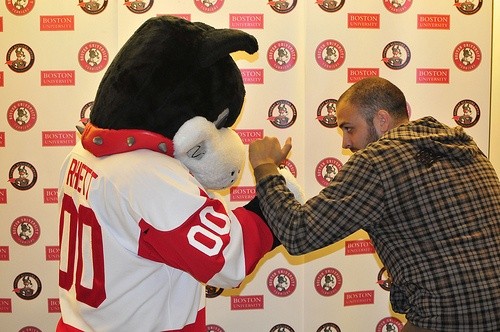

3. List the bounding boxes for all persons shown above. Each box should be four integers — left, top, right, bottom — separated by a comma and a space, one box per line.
249, 77, 500, 332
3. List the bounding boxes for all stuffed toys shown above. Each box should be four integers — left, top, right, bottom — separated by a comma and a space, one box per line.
56, 14, 304, 332
